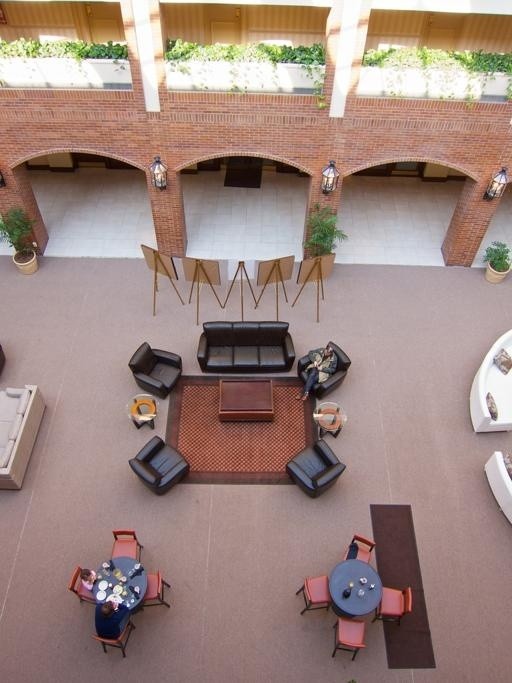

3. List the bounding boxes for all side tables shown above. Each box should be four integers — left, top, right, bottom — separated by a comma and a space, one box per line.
312, 401, 347, 438
126, 394, 162, 430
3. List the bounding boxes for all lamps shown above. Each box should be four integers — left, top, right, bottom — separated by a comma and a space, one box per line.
483, 167, 511, 201
321, 161, 341, 194
149, 155, 168, 190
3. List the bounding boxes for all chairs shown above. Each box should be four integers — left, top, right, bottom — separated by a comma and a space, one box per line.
67, 529, 169, 659
129, 342, 182, 400
296, 534, 413, 662
298, 341, 352, 401
286, 439, 347, 498
129, 435, 190, 495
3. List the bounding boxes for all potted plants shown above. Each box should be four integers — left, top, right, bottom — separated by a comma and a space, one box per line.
484, 240, 512, 283
1, 208, 40, 275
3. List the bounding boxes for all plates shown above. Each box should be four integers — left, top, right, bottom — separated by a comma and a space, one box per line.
96, 580, 123, 611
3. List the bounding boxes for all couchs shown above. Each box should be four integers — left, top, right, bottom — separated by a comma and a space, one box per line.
1, 385, 46, 490
198, 321, 296, 372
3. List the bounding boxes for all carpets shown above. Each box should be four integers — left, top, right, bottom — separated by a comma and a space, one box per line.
166, 376, 318, 484
369, 503, 437, 670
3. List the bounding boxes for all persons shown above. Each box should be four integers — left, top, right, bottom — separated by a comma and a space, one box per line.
94, 600, 131, 640
295, 346, 339, 401
79, 568, 98, 591
